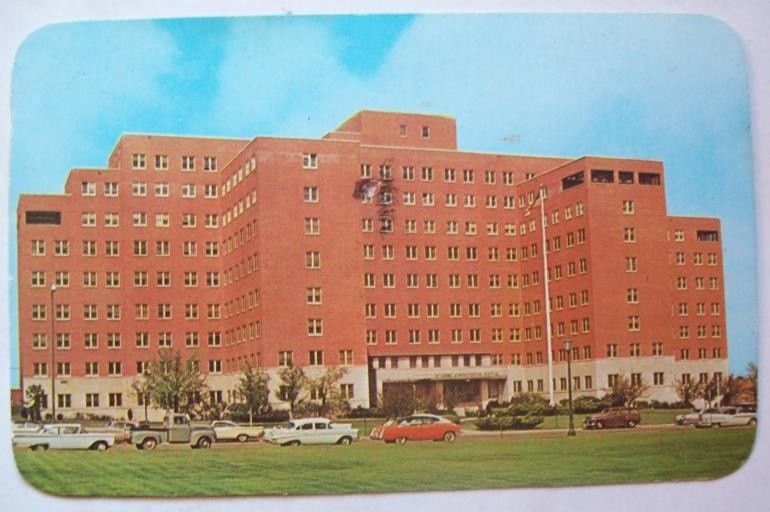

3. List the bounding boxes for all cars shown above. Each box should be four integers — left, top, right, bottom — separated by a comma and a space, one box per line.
12, 423, 115, 452
263, 417, 360, 447
676, 401, 757, 427
82, 419, 141, 445
12, 421, 65, 435
370, 413, 461, 446
206, 418, 266, 442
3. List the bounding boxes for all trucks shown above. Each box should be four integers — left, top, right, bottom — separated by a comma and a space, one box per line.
129, 414, 217, 451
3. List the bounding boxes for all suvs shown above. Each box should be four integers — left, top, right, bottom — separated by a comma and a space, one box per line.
584, 406, 641, 430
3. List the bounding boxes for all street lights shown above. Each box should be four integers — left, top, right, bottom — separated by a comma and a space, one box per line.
50, 282, 58, 422
563, 336, 578, 438
522, 181, 556, 407
